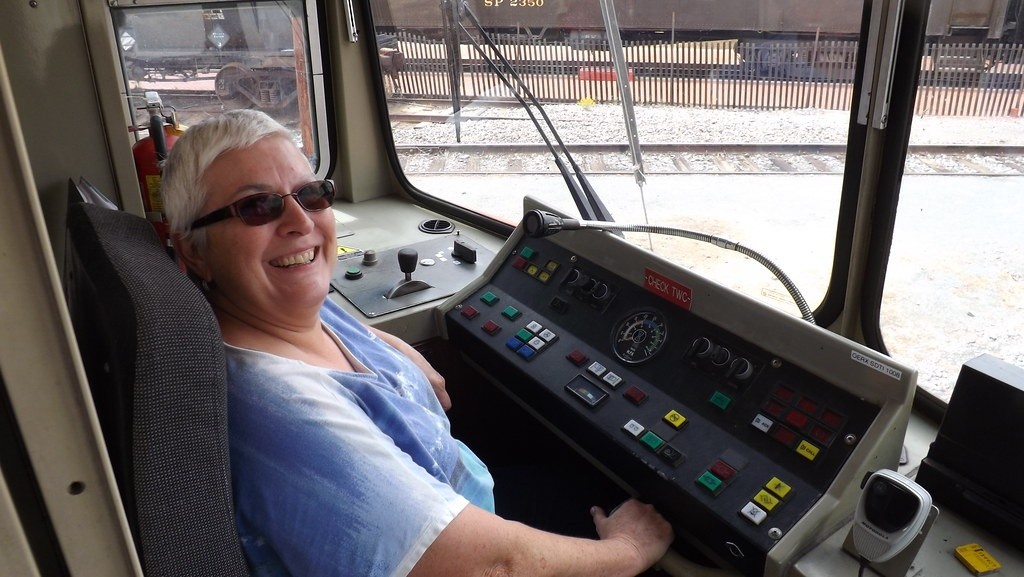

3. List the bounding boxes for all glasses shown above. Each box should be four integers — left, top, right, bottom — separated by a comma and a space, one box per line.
185, 179, 336, 232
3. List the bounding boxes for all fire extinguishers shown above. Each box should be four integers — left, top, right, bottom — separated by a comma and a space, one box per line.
122, 91, 186, 256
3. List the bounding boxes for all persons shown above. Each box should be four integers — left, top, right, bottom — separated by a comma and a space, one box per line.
162, 109, 674, 577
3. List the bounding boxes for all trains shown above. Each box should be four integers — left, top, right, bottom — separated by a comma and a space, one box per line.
114, 0, 402, 115
372, 0, 1024, 77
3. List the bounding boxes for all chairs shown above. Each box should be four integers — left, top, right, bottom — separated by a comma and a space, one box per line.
65, 202, 252, 577
